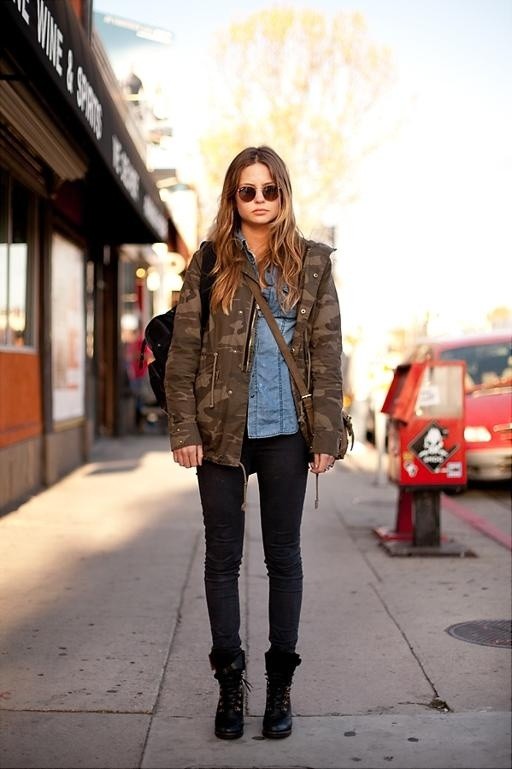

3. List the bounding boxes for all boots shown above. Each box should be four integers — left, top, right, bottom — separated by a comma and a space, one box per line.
208, 645, 254, 739
261, 651, 303, 739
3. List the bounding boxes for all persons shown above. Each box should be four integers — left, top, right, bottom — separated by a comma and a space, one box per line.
163, 142, 343, 740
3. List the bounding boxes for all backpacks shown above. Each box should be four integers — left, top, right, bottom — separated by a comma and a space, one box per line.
143, 240, 217, 413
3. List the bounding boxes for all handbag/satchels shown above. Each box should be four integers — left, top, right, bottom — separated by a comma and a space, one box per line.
298, 393, 355, 461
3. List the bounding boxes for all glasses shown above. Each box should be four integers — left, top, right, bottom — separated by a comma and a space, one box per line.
235, 185, 279, 202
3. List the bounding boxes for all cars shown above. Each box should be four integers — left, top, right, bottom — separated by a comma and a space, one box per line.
409, 334, 512, 496
